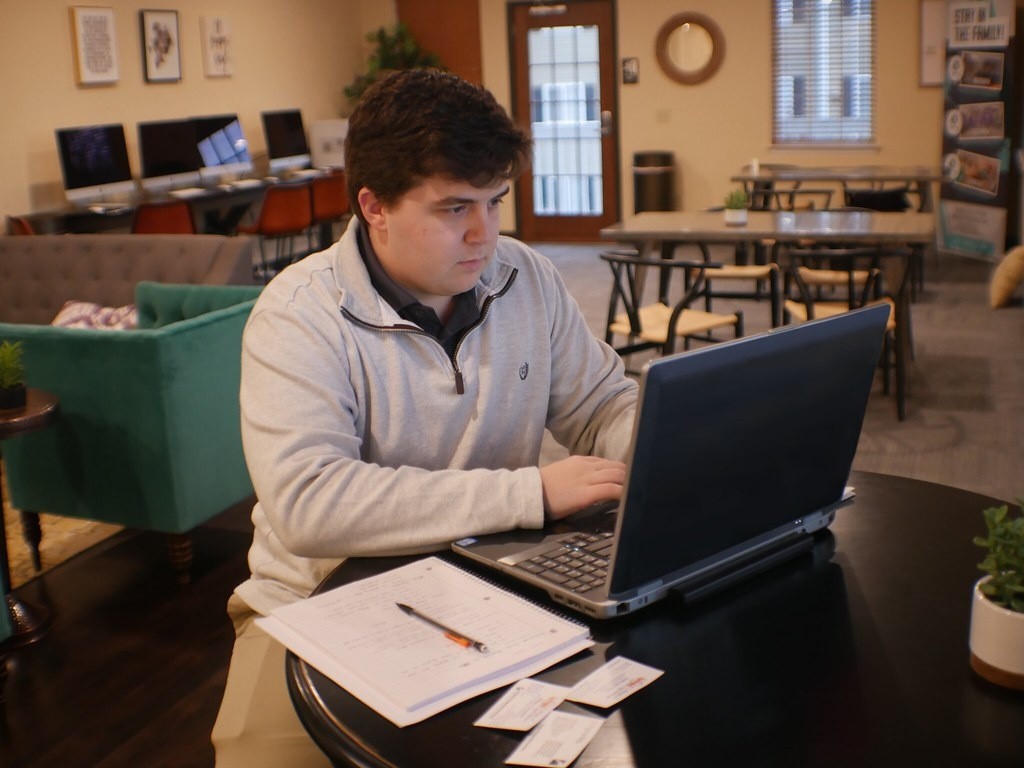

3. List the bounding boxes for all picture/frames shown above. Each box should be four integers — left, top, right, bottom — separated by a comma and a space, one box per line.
141, 9, 183, 84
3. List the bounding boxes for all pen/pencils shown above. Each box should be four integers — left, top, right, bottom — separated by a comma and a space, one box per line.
395, 603, 487, 652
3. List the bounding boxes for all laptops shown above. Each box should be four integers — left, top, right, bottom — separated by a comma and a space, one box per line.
449, 303, 892, 620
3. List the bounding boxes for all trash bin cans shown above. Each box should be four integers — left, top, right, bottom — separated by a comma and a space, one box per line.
632, 151, 673, 215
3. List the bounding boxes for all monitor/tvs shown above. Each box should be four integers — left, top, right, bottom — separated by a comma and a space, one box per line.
54, 109, 312, 202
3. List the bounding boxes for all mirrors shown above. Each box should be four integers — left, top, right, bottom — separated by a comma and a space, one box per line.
656, 9, 726, 86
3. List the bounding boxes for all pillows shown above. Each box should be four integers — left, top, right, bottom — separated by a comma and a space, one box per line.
989, 243, 1024, 307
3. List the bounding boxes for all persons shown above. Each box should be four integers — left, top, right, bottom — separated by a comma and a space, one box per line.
211, 67, 640, 768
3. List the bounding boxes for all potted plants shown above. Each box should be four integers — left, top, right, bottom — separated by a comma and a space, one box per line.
724, 189, 748, 225
965, 501, 1024, 690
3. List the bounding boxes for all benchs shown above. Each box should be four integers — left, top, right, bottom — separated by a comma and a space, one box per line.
3, 229, 256, 329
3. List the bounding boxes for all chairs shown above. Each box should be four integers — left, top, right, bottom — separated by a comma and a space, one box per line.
0, 282, 272, 585
130, 200, 198, 236
597, 160, 929, 423
235, 182, 314, 281
301, 173, 353, 261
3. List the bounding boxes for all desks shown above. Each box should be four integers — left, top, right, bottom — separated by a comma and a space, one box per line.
60, 164, 346, 236
600, 209, 935, 420
728, 168, 933, 292
283, 446, 1024, 766
1, 386, 63, 651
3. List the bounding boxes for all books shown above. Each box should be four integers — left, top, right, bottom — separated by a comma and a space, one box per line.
252, 557, 595, 728
